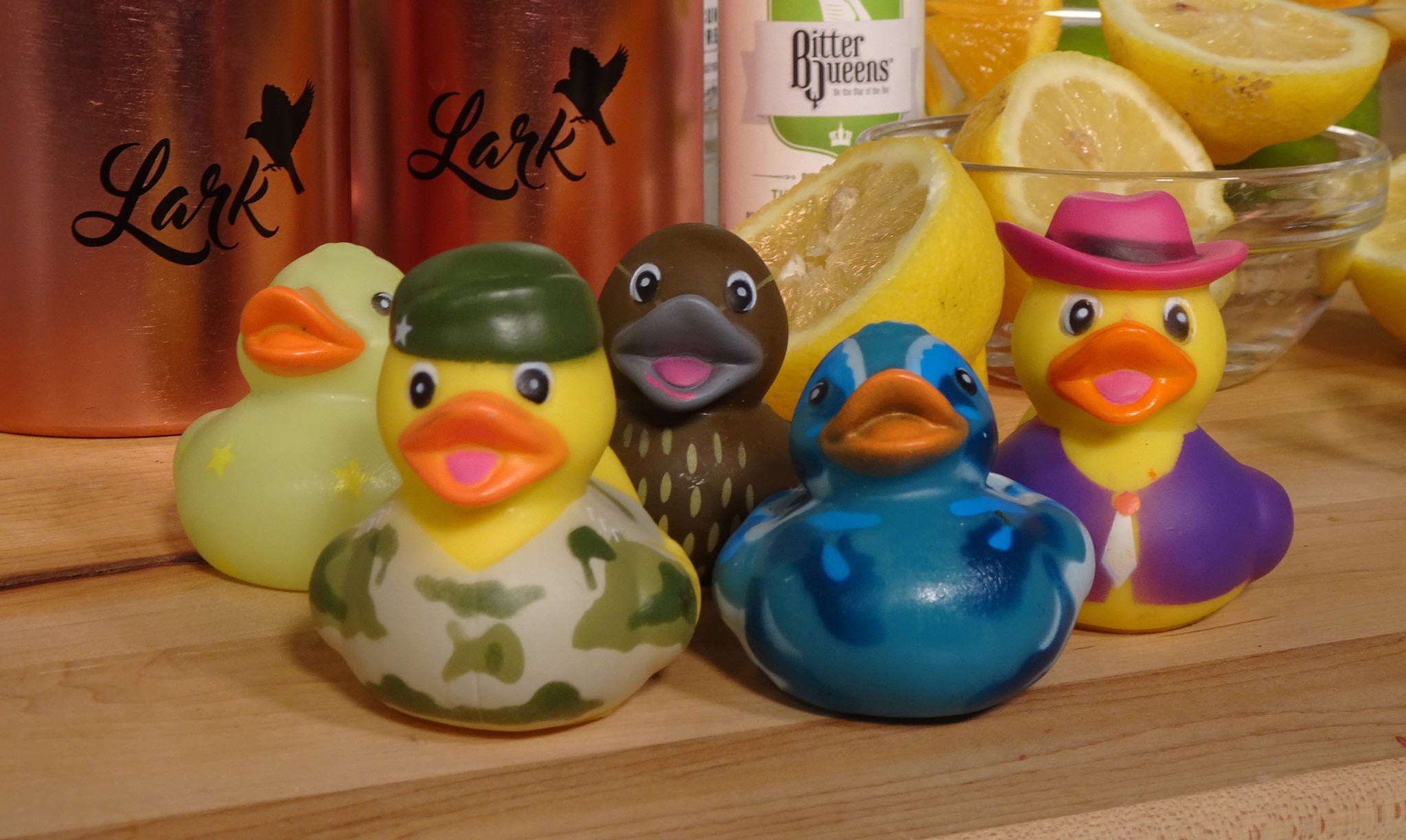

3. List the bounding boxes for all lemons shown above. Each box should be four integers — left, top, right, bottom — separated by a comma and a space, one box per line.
918, 1, 1389, 332
732, 134, 1006, 426
1349, 150, 1406, 343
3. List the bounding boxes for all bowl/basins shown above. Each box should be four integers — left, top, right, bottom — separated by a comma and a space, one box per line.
853, 111, 1392, 394
